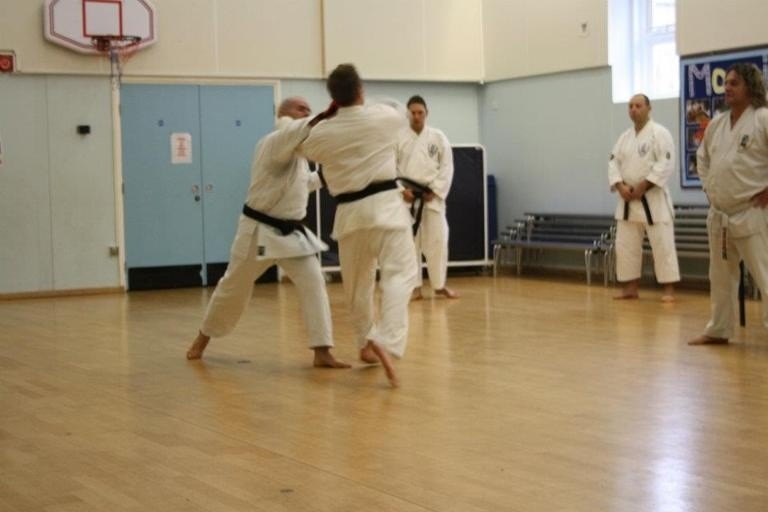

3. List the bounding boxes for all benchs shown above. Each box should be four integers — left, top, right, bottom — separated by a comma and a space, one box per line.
491, 203, 759, 299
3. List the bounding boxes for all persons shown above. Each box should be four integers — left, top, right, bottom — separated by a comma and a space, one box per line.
186, 97, 352, 369
278, 63, 412, 388
394, 95, 461, 299
607, 93, 682, 302
687, 98, 712, 121
686, 62, 767, 345
